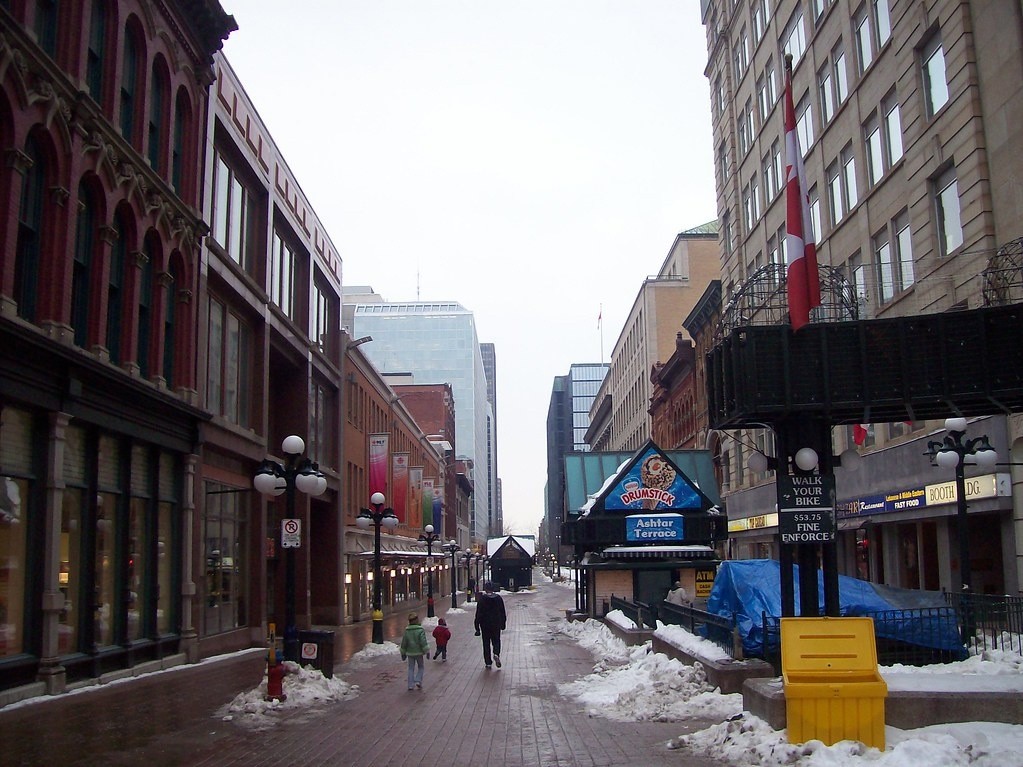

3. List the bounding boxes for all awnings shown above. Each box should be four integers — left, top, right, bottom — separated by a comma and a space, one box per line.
357, 551, 445, 559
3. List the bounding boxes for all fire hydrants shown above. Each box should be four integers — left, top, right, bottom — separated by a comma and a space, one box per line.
264, 647, 289, 702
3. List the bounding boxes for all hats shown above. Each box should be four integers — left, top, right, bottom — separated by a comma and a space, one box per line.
408, 611, 419, 624
670, 581, 678, 591
438, 618, 446, 625
485, 581, 494, 591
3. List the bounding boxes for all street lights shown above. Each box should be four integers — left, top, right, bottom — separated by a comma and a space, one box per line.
556, 516, 561, 578
462, 548, 489, 604
927, 418, 998, 647
416, 525, 442, 618
254, 436, 327, 664
444, 540, 463, 609
357, 492, 400, 645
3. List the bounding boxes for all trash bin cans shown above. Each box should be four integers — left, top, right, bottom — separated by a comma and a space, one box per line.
779, 616, 888, 754
297, 629, 335, 679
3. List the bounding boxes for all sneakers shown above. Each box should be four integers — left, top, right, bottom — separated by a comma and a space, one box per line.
494, 654, 501, 668
484, 665, 491, 668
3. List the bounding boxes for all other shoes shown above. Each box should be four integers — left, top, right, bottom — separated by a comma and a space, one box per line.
443, 659, 447, 662
408, 687, 413, 690
416, 682, 422, 688
433, 656, 436, 660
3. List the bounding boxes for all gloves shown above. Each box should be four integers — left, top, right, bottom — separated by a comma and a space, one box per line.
686, 599, 690, 603
402, 655, 406, 661
426, 653, 430, 659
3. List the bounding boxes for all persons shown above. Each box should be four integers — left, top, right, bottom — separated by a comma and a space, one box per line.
666, 581, 689, 605
474, 582, 506, 670
469, 576, 475, 594
432, 618, 451, 663
399, 612, 430, 691
